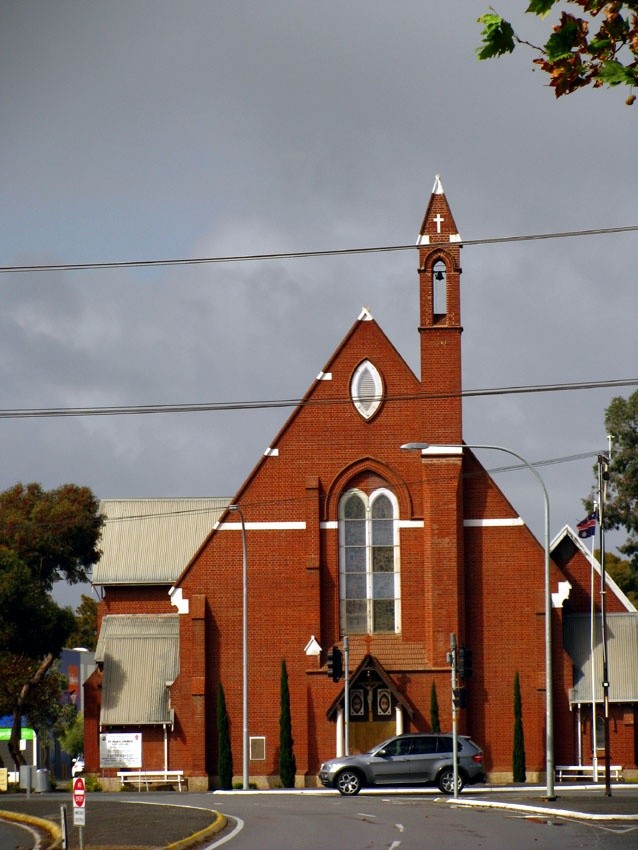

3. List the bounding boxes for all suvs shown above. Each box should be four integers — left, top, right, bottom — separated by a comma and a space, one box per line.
319, 732, 488, 795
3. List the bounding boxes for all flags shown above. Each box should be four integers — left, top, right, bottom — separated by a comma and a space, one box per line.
576, 511, 596, 538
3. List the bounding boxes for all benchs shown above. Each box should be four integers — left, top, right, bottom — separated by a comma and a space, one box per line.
117, 770, 185, 792
555, 766, 622, 781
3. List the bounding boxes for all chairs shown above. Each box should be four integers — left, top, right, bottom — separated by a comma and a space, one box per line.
398, 741, 416, 754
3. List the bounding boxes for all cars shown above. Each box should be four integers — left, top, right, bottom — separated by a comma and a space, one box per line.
72, 755, 85, 776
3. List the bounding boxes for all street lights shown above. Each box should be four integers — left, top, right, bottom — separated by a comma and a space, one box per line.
401, 442, 555, 797
230, 505, 249, 791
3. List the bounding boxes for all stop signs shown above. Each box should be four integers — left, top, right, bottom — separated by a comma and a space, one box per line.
73, 776, 85, 807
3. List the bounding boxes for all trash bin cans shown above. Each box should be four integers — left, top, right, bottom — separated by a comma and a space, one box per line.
35, 768, 49, 793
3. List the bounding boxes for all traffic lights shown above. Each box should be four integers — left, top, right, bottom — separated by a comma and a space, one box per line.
326, 647, 342, 681
459, 648, 474, 677
453, 685, 468, 709
